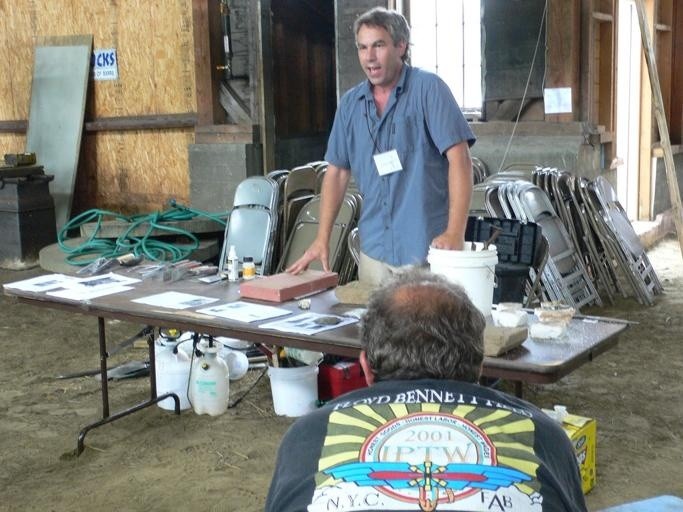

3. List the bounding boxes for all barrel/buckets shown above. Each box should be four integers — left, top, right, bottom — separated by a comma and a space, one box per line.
189, 349, 230, 416
155, 332, 192, 412
263, 363, 321, 417
427, 240, 500, 317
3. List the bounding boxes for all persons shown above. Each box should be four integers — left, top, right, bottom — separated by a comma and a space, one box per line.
263, 268, 589, 510
282, 6, 479, 287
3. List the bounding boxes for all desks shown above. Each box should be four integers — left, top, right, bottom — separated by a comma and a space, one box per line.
3, 264, 630, 461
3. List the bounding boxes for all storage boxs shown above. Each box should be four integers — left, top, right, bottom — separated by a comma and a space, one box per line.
540, 407, 596, 495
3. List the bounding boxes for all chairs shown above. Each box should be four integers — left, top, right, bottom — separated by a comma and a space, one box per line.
213, 157, 662, 315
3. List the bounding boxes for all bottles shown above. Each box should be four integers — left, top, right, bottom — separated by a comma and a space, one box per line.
227, 246, 239, 281
243, 257, 255, 279
